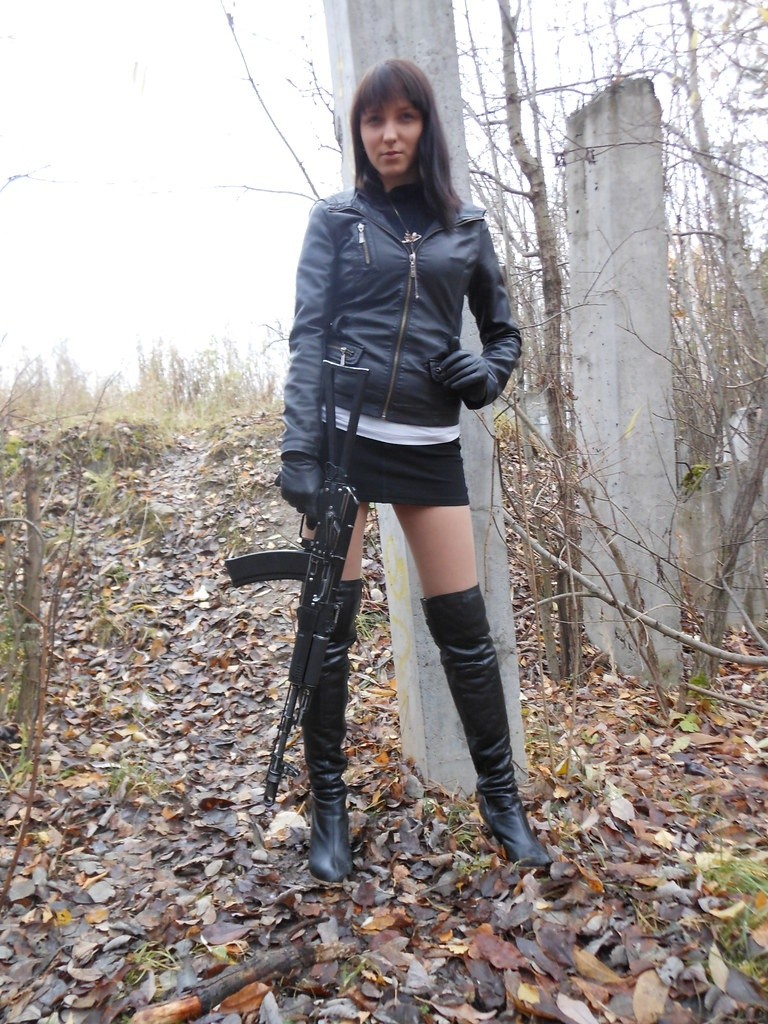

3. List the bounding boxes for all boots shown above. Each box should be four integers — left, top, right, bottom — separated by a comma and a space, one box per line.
297, 579, 363, 883
420, 582, 554, 870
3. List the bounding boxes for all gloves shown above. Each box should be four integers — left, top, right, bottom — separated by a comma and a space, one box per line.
281, 452, 327, 530
437, 349, 486, 407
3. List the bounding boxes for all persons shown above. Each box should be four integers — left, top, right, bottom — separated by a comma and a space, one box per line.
277, 60, 552, 879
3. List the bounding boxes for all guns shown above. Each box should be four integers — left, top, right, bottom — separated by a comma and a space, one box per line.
220, 358, 374, 810
